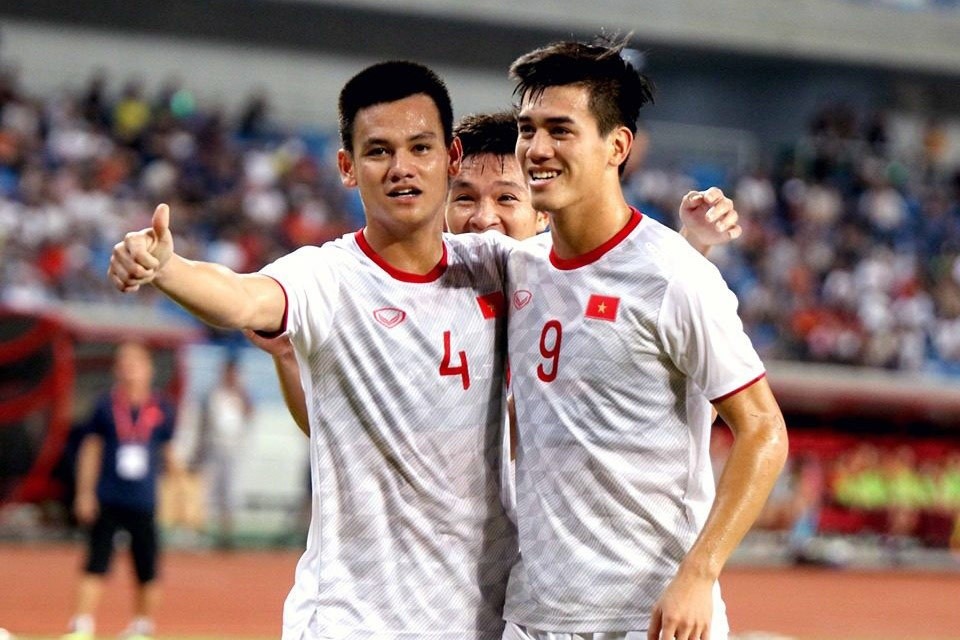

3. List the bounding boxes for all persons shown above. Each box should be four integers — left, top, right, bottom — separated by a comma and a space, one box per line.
0, 63, 960, 384
443, 33, 788, 640
241, 107, 550, 514
60, 336, 189, 640
107, 57, 741, 640
190, 356, 254, 555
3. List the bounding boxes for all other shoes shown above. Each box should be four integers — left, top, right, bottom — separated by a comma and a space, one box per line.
124, 619, 153, 640
65, 613, 96, 640
